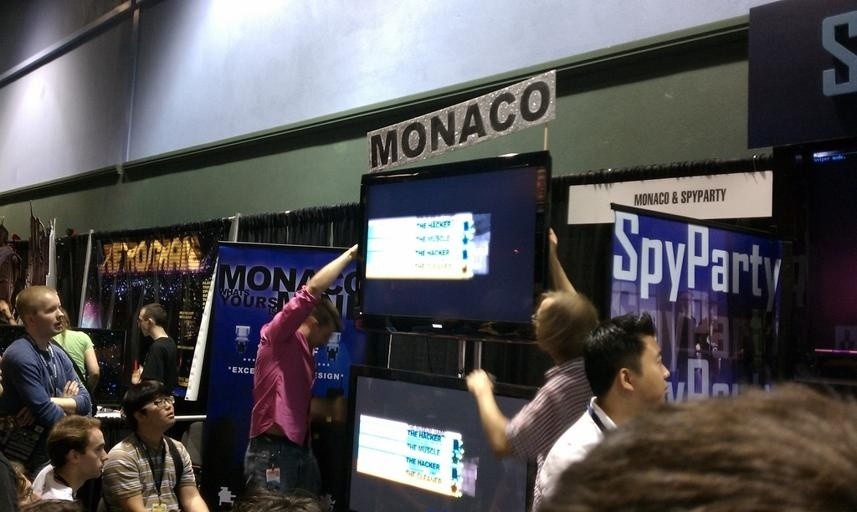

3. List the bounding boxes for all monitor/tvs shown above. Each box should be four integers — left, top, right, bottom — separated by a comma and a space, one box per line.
342, 364, 542, 512
353, 149, 552, 344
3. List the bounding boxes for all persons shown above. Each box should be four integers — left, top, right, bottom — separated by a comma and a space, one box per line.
735, 318, 759, 382
700, 302, 721, 358
230, 487, 338, 511
538, 312, 673, 506
464, 226, 602, 510
4, 284, 211, 511
241, 239, 360, 491
537, 383, 855, 511
